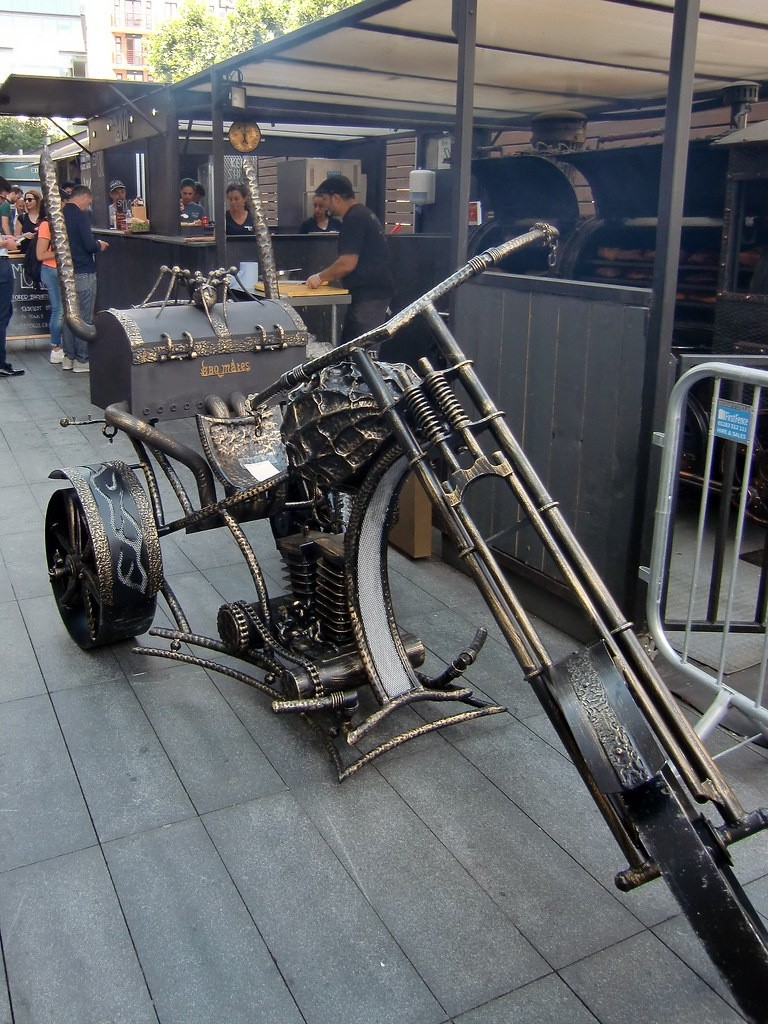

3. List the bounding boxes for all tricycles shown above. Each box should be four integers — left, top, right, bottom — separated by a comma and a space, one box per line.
37, 144, 768, 1024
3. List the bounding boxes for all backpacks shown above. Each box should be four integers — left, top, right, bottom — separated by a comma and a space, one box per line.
22, 219, 51, 283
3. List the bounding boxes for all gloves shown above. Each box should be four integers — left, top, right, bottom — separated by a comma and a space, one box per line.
305, 273, 322, 289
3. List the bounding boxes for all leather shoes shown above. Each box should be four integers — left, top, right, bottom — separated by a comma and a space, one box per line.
0, 363, 25, 377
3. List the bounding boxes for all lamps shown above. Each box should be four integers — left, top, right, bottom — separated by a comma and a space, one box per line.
224, 69, 246, 110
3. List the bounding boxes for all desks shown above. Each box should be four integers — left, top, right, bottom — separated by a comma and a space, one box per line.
231, 280, 352, 349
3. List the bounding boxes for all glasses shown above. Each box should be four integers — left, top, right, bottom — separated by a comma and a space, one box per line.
24, 198, 35, 203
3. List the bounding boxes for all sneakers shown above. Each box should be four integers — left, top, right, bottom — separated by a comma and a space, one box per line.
50, 349, 64, 363
62, 356, 74, 370
72, 359, 90, 372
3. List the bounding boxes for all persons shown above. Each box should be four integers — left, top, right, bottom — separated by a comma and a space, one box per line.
316, 174, 395, 364
225, 183, 255, 235
300, 194, 342, 235
2, 174, 207, 371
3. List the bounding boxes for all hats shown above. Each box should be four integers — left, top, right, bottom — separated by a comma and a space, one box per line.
109, 179, 125, 192
313, 175, 353, 194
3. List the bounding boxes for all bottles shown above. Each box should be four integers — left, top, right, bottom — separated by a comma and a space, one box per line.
125, 210, 133, 230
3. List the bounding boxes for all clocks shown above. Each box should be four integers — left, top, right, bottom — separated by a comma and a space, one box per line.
229, 118, 261, 153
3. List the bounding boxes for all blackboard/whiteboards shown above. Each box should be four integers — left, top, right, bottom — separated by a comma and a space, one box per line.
5, 254, 62, 340
